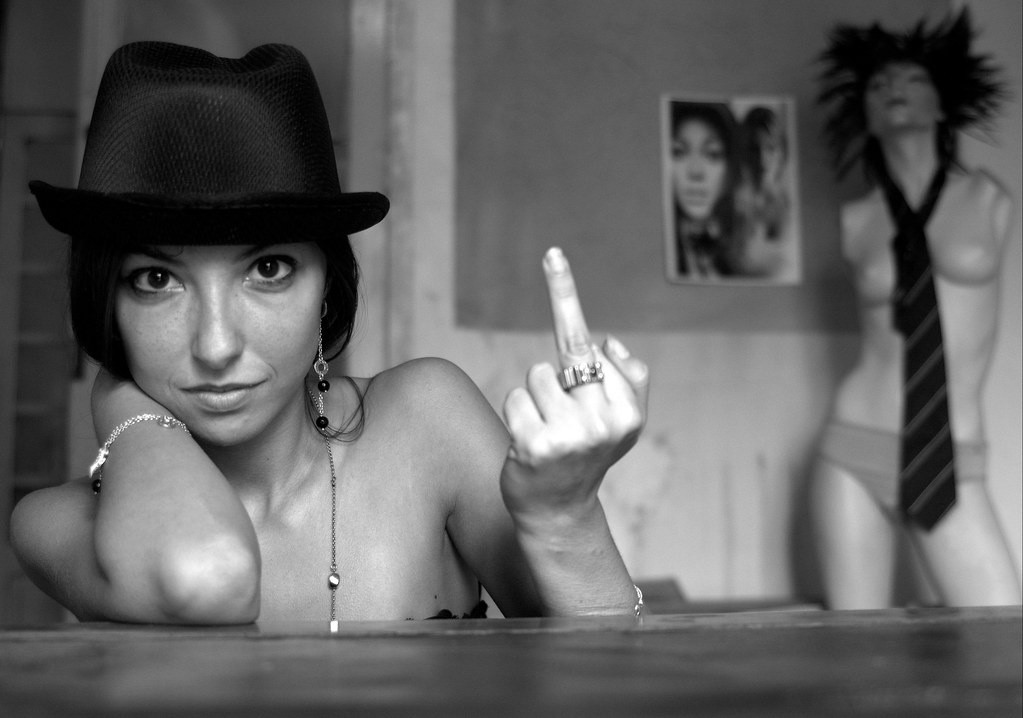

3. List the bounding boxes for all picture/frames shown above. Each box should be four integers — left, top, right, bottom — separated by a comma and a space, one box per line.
660, 92, 801, 289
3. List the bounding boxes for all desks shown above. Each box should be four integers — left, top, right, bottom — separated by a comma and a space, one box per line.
0, 608, 1023, 718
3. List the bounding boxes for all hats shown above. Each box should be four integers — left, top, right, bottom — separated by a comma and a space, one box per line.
28, 40, 391, 245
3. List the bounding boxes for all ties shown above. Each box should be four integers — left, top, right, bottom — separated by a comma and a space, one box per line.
881, 158, 958, 533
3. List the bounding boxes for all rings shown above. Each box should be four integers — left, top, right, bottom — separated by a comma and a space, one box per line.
558, 362, 605, 389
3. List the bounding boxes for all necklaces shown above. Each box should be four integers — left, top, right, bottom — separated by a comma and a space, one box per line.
309, 387, 339, 619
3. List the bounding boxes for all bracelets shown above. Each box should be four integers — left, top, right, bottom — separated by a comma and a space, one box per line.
89, 415, 192, 496
634, 584, 643, 615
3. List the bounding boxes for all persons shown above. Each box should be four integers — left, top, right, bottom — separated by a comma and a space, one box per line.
10, 37, 651, 626
669, 102, 792, 281
810, 9, 1019, 610
